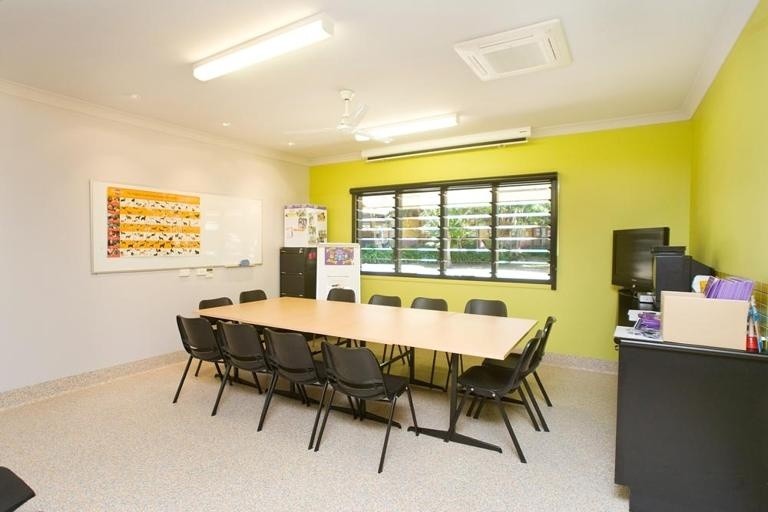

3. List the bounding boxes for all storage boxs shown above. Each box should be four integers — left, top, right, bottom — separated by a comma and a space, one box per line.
659, 290, 749, 353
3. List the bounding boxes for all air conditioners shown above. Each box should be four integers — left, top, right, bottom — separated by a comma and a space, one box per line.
360, 125, 532, 167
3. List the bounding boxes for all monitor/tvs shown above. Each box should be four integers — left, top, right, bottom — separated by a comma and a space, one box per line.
611, 227, 670, 296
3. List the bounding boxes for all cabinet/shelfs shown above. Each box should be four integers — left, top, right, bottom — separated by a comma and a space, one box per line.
280, 247, 316, 300
317, 243, 360, 306
613, 306, 768, 512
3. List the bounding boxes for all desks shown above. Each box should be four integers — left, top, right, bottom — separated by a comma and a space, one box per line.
193, 296, 538, 455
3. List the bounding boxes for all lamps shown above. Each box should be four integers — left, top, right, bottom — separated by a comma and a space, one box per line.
192, 17, 334, 79
346, 118, 459, 143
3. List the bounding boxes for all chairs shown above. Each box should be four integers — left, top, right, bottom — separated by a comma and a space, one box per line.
194, 296, 232, 386
410, 298, 449, 310
464, 298, 504, 316
257, 327, 357, 451
173, 313, 234, 403
370, 294, 400, 306
237, 289, 266, 302
446, 330, 545, 466
209, 321, 306, 432
316, 340, 419, 478
328, 289, 354, 304
466, 316, 559, 437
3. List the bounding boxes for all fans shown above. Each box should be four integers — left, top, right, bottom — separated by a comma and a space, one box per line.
293, 99, 393, 147
